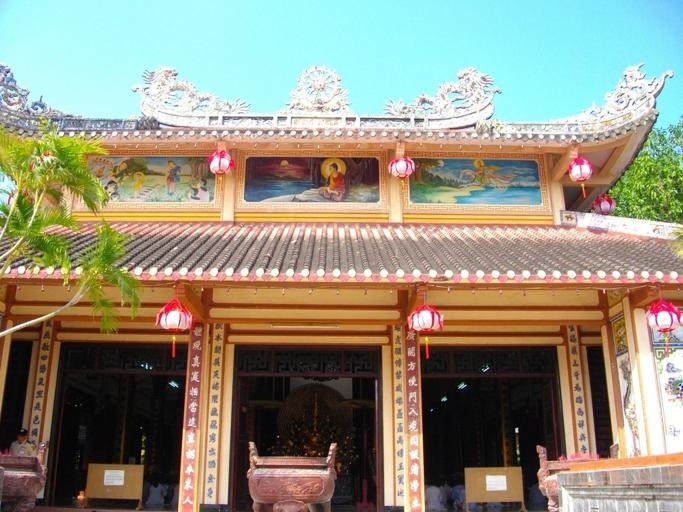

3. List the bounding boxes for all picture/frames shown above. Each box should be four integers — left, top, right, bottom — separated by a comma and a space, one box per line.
403, 146, 554, 215
230, 141, 392, 214
76, 142, 224, 212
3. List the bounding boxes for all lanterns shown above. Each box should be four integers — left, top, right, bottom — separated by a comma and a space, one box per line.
592, 194, 615, 215
407, 304, 444, 360
387, 157, 416, 191
30, 152, 61, 179
208, 151, 236, 188
154, 298, 193, 358
567, 157, 594, 201
646, 300, 682, 355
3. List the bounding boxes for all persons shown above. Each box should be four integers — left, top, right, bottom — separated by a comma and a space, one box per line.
424, 480, 546, 512
264, 391, 358, 475
10, 429, 37, 455
143, 479, 178, 510
